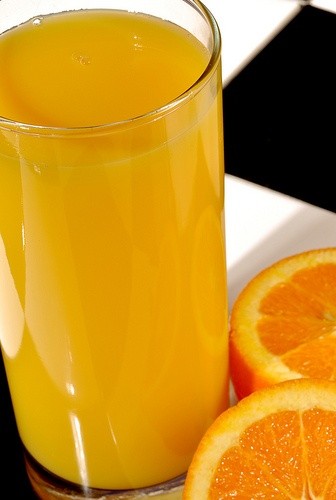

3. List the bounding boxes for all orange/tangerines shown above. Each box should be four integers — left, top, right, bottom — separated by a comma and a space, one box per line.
229, 247, 336, 402
182, 377, 336, 500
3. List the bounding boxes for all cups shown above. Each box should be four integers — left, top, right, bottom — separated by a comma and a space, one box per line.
0, 0, 231, 500
